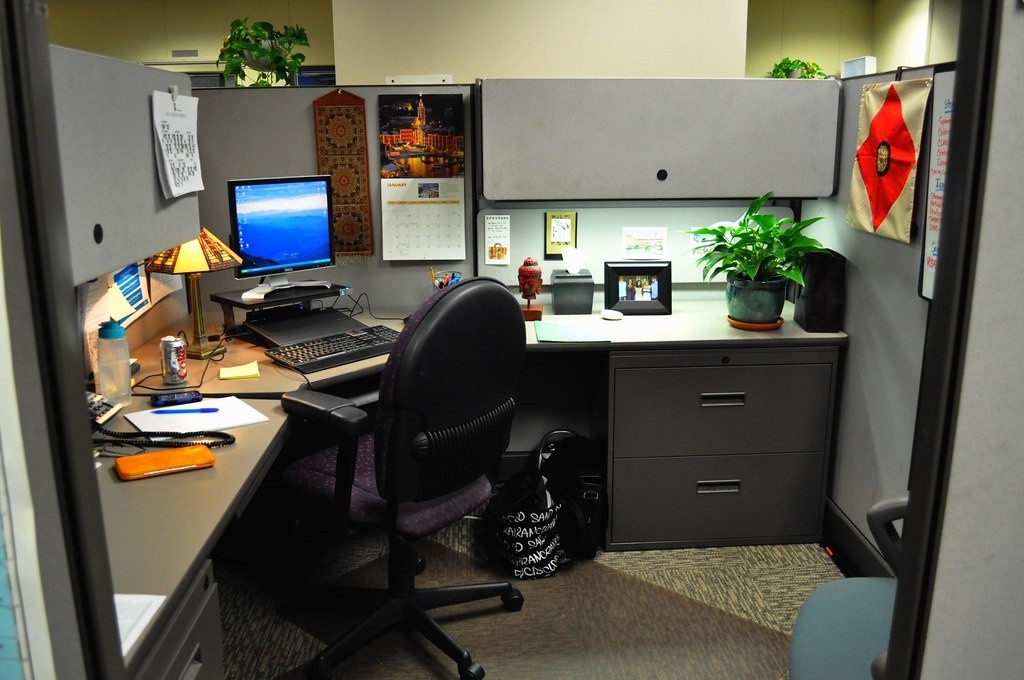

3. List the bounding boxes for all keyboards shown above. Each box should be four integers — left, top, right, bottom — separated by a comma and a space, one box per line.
263, 325, 402, 374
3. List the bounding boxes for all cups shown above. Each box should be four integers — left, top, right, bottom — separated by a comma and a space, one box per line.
432, 271, 463, 295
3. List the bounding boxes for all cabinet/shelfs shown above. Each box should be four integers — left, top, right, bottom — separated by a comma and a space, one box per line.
196, 291, 850, 553
82, 315, 313, 680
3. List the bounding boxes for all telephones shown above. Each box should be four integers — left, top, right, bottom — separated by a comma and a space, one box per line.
86, 391, 123, 433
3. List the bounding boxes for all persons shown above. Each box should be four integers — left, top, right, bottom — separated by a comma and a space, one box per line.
518, 256, 542, 299
619, 276, 627, 301
650, 275, 658, 300
626, 278, 635, 300
641, 280, 651, 300
634, 280, 643, 301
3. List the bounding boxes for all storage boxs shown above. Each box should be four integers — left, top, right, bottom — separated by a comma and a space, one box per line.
840, 56, 877, 79
551, 269, 594, 315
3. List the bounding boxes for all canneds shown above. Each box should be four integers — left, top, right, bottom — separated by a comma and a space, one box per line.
160, 335, 187, 384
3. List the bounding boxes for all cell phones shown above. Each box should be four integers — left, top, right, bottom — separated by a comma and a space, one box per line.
151, 390, 203, 407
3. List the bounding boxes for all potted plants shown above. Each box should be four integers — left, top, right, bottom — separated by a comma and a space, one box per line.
217, 16, 310, 88
688, 191, 833, 330
766, 58, 825, 80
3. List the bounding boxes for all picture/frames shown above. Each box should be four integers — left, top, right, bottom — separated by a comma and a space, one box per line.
604, 261, 671, 314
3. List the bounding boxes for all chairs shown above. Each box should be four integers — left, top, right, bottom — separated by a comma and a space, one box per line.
259, 278, 525, 680
789, 498, 908, 679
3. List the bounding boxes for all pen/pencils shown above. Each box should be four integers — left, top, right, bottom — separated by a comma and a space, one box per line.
434, 272, 461, 289
150, 408, 219, 415
430, 266, 436, 283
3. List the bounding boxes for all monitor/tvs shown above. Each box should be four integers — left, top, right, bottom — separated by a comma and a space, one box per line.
227, 174, 338, 302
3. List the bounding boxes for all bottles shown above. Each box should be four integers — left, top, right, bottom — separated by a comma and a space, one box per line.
96, 321, 132, 406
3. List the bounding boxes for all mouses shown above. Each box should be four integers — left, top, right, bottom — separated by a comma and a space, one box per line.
403, 315, 414, 324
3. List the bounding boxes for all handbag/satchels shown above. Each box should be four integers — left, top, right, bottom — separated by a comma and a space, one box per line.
541, 435, 607, 561
476, 471, 564, 579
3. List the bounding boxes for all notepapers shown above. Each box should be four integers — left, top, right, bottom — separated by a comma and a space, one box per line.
219, 361, 261, 379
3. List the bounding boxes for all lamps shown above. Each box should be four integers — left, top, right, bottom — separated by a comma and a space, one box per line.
145, 227, 243, 359
